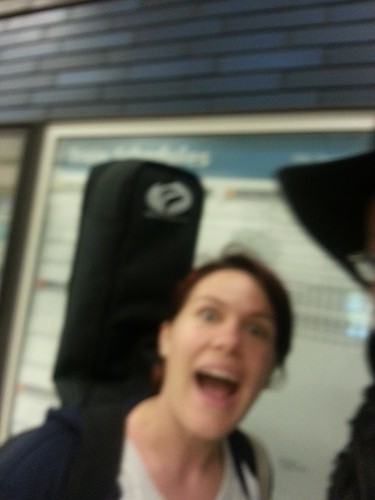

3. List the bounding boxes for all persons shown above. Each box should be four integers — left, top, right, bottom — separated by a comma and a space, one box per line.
0, 243, 293, 499
